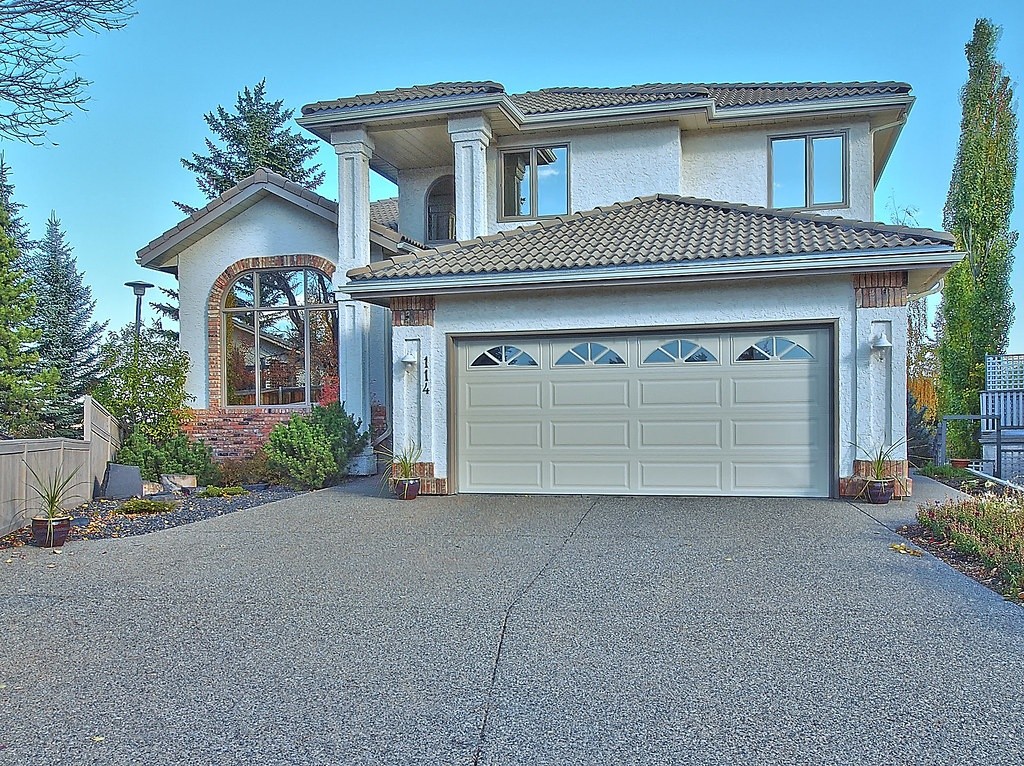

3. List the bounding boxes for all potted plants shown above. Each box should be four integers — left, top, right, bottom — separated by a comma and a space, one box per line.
373, 440, 423, 500
845, 436, 934, 504
1, 455, 97, 547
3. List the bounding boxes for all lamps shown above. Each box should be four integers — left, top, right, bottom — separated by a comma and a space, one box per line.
401, 350, 416, 368
872, 333, 892, 360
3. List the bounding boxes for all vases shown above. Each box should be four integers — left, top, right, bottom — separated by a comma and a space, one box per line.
950, 460, 970, 468
